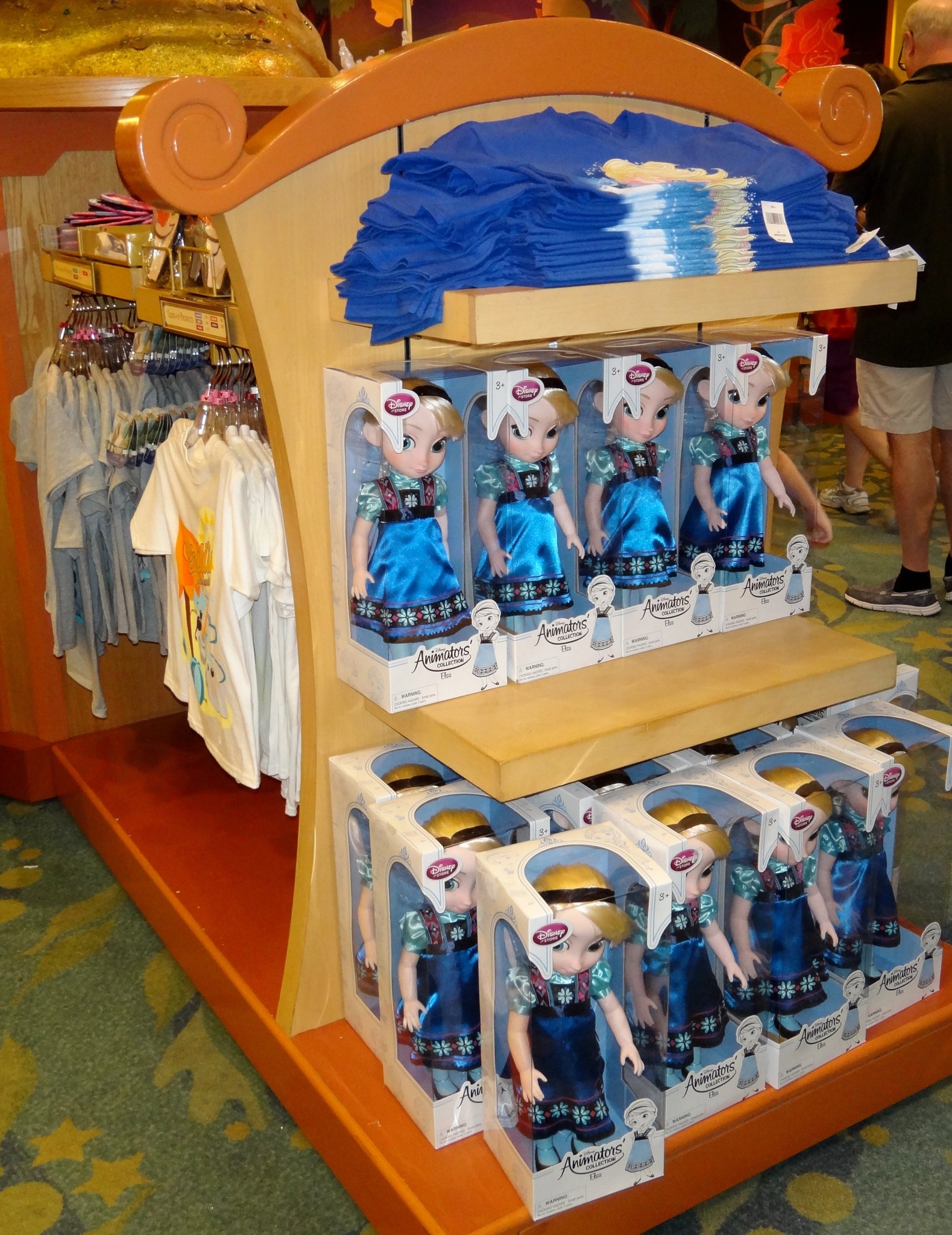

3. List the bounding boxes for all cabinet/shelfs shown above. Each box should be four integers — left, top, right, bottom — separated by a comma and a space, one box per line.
0, 0, 952, 1235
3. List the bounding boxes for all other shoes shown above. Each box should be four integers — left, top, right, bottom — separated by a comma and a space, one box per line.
943, 575, 952, 601
844, 575, 942, 617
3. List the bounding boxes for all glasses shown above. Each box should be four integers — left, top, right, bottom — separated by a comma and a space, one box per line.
897, 30, 913, 72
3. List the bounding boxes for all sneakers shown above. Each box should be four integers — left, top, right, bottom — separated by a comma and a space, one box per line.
820, 483, 871, 514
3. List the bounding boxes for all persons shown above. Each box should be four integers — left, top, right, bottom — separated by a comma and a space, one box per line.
355, 716, 916, 1172
777, 0, 952, 617
579, 352, 677, 609
844, 0, 952, 617
820, 63, 940, 515
355, 764, 446, 997
350, 377, 472, 660
507, 862, 645, 1172
776, 449, 833, 549
394, 807, 505, 1100
677, 343, 795, 587
723, 766, 838, 1040
472, 361, 585, 635
624, 798, 747, 1092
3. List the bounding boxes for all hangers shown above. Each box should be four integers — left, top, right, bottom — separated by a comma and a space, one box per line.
170, 342, 272, 468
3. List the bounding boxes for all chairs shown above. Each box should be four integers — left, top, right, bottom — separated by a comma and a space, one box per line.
38, 294, 139, 400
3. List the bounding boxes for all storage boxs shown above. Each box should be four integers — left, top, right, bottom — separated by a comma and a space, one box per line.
81, 226, 157, 269
328, 325, 952, 1221
38, 224, 83, 258
